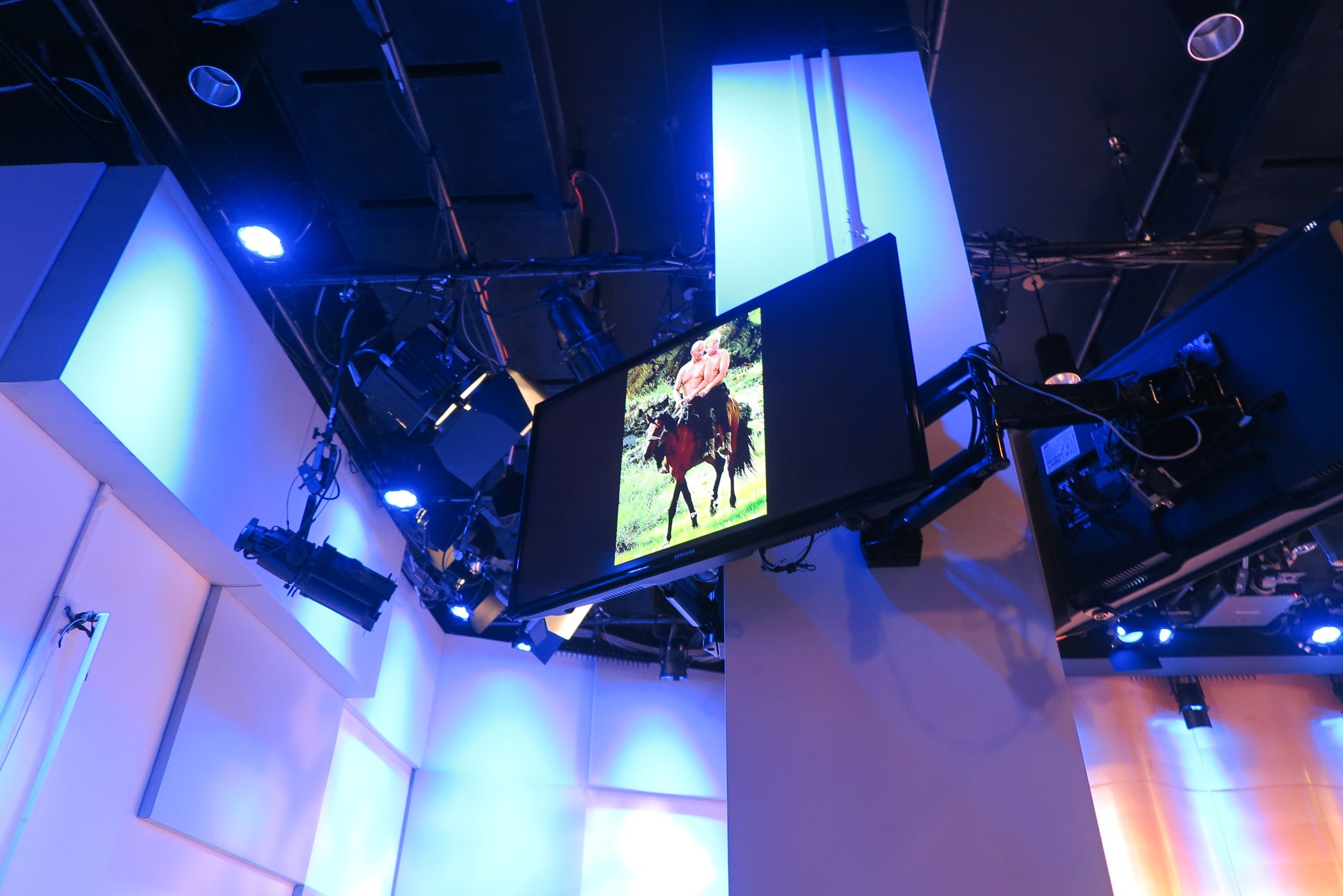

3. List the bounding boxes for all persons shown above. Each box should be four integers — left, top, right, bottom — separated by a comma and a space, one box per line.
672, 331, 733, 460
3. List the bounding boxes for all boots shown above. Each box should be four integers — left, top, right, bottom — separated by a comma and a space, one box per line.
702, 438, 715, 461
720, 432, 733, 455
660, 456, 669, 474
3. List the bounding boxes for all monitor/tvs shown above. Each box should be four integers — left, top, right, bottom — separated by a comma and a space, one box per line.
509, 233, 930, 623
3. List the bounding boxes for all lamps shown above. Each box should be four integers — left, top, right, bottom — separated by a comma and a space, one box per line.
1298, 608, 1343, 648
187, 24, 259, 108
537, 275, 624, 382
1136, 604, 1175, 646
1168, 673, 1214, 731
1022, 273, 1085, 386
230, 278, 401, 633
659, 622, 692, 681
225, 191, 307, 268
1164, 0, 1246, 63
447, 576, 487, 622
511, 619, 534, 652
1111, 614, 1145, 645
378, 456, 423, 513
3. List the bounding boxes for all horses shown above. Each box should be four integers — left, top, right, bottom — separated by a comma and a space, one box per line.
643, 396, 759, 545
